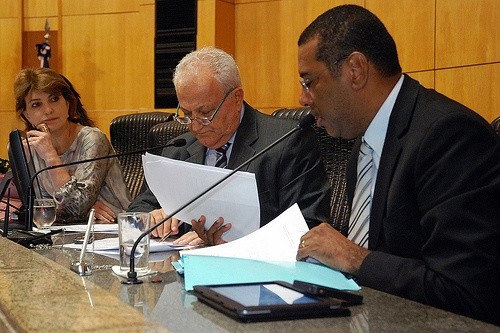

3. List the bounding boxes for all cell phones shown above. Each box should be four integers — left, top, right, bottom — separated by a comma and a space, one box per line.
293, 281, 364, 303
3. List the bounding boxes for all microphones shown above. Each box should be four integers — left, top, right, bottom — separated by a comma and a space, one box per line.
12, 139, 187, 249
122, 113, 317, 285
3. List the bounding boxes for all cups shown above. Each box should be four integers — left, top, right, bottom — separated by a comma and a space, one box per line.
117, 212, 151, 274
33, 199, 56, 228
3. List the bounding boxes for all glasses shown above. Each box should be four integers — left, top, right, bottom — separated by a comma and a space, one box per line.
175, 85, 235, 125
299, 54, 350, 91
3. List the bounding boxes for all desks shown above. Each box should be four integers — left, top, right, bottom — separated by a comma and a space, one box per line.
0, 227, 500, 333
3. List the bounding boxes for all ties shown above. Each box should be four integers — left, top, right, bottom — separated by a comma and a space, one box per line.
214, 142, 230, 169
346, 139, 375, 251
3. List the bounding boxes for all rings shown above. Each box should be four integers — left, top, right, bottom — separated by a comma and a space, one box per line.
41, 124, 51, 133
301, 239, 306, 247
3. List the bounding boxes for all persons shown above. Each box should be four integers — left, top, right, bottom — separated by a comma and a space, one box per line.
7, 66, 133, 225
296, 3, 500, 325
126, 46, 331, 264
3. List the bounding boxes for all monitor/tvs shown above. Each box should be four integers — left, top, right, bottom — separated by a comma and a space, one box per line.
0, 129, 39, 231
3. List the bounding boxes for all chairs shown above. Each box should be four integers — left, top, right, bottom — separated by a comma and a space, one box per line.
271, 107, 358, 235
490, 115, 500, 135
109, 112, 190, 201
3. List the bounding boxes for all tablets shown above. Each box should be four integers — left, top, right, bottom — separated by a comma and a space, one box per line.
192, 281, 340, 322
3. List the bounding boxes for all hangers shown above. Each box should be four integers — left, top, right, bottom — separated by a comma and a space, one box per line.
35, 18, 52, 68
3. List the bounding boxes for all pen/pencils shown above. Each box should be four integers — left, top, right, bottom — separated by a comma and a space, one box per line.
5, 188, 11, 237
158, 220, 183, 243
80, 209, 96, 262
